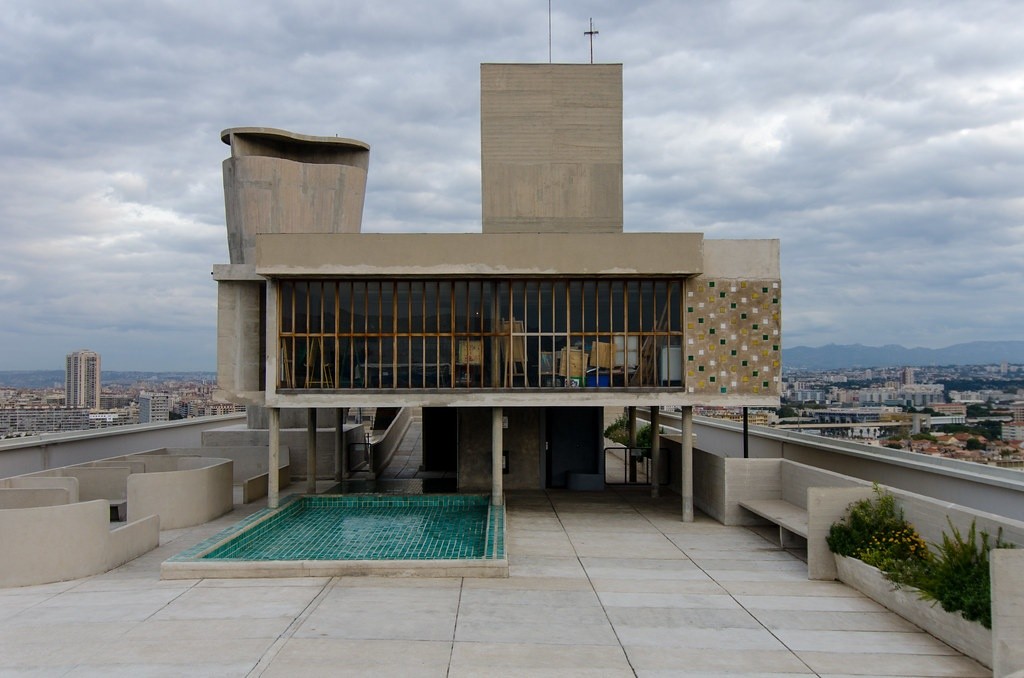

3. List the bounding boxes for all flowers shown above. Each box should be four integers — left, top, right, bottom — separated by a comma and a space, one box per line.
826, 480, 1018, 630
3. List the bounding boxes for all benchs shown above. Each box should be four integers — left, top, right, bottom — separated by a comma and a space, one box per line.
739, 499, 807, 564
109, 499, 127, 522
